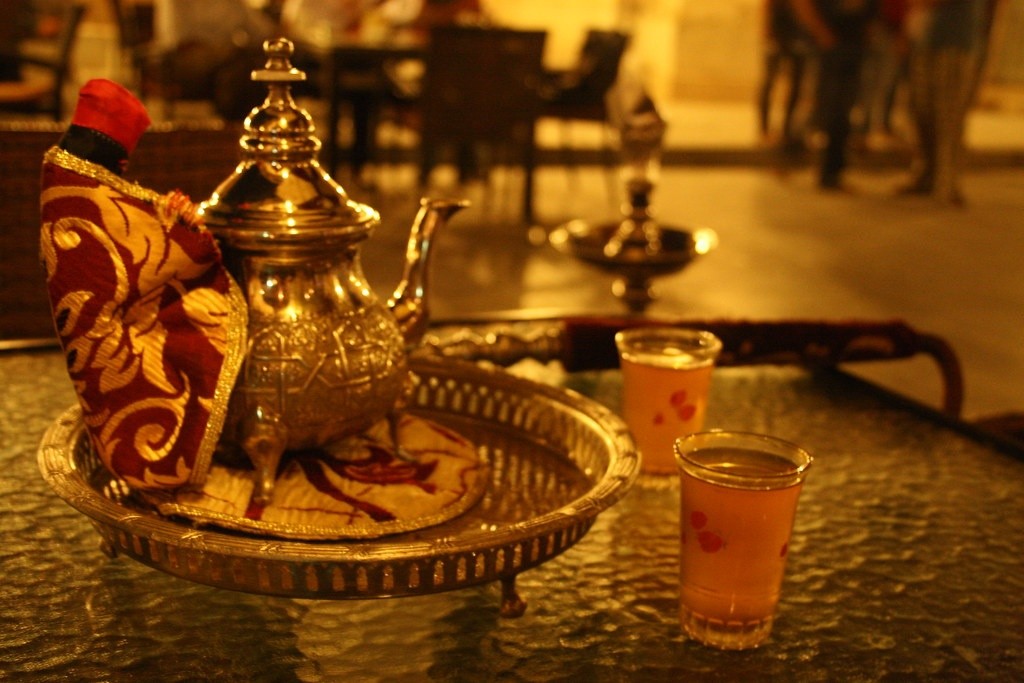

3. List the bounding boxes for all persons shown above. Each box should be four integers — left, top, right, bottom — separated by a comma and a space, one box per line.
883, 1, 996, 207
790, 0, 880, 204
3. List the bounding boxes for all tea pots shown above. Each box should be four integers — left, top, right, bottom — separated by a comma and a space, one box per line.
170, 36, 470, 507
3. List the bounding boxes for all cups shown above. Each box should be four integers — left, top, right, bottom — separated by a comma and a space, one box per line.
671, 428, 814, 652
614, 325, 722, 475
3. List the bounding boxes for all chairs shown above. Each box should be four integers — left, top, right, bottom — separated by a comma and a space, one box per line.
503, 30, 632, 213
412, 20, 546, 225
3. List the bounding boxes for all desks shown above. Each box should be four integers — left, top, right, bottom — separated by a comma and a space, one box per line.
0, 311, 1024, 683
0, 111, 248, 340
